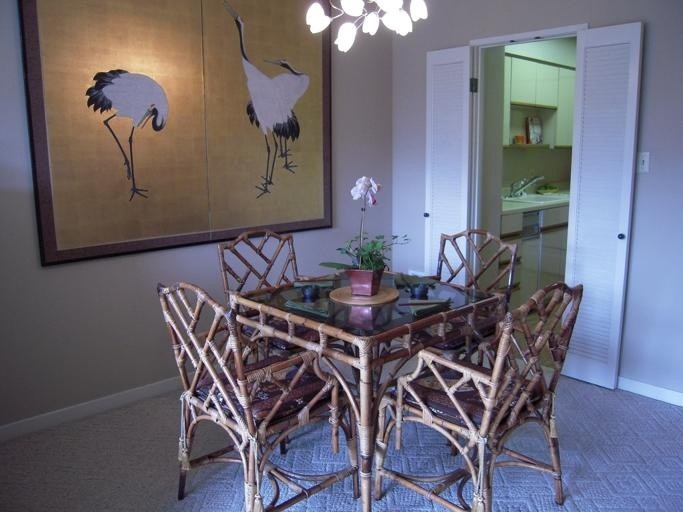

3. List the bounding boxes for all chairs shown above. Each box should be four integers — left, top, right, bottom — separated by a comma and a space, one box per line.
373, 282, 583, 512
157, 282, 360, 512
393, 229, 517, 445
217, 228, 337, 444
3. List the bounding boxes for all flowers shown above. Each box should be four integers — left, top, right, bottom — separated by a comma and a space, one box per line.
336, 176, 408, 269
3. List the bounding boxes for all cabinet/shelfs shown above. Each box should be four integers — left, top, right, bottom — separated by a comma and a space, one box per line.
504, 54, 575, 149
500, 205, 523, 312
537, 206, 569, 289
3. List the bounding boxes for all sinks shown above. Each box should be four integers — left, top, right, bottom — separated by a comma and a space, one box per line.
503, 192, 570, 203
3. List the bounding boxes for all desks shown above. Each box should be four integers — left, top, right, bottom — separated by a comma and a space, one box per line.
230, 271, 507, 512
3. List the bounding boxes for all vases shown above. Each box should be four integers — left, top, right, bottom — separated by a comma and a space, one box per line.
345, 269, 384, 296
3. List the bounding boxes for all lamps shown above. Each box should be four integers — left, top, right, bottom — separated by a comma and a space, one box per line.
304, 1, 429, 54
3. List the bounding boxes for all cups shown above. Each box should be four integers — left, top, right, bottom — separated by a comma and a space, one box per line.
411, 284, 429, 297
302, 285, 322, 299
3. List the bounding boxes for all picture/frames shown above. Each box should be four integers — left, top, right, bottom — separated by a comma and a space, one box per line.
18, 1, 333, 267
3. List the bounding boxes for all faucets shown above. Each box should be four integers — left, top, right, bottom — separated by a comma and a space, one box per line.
511, 175, 545, 196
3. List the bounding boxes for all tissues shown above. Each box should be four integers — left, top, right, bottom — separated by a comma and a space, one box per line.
536, 181, 559, 193
